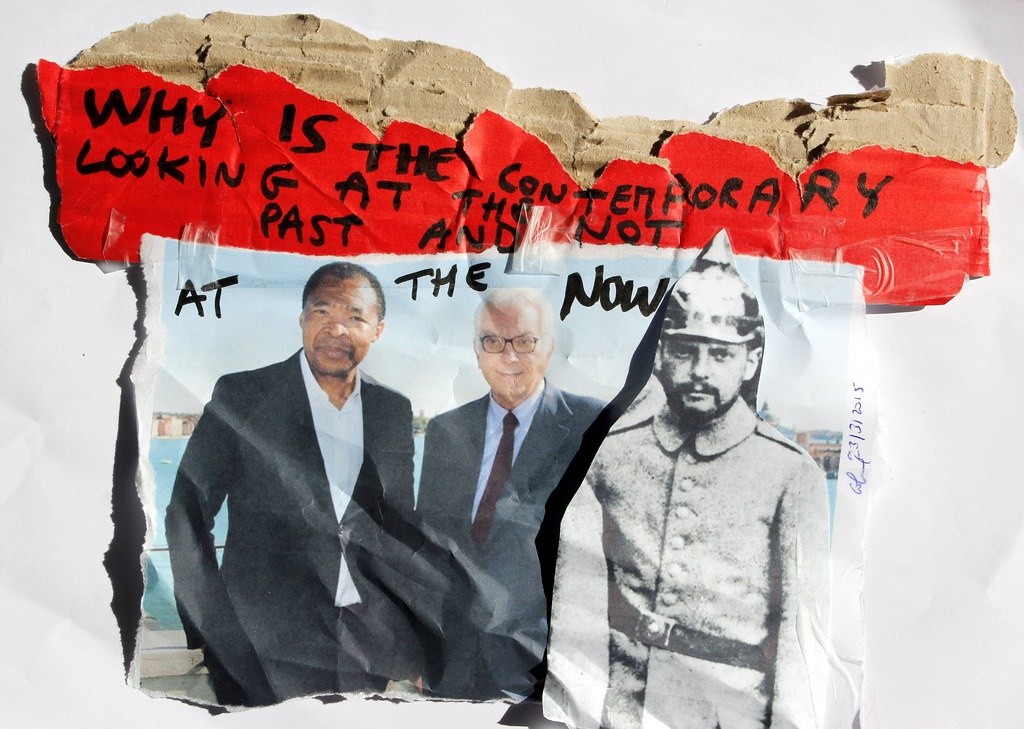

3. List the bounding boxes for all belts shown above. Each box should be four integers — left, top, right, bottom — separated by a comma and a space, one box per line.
606, 558, 768, 675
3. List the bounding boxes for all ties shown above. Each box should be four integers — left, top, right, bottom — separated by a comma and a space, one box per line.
471, 410, 520, 552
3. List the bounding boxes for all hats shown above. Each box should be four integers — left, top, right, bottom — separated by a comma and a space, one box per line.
661, 259, 765, 343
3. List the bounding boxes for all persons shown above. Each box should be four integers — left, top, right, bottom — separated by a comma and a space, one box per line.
160, 259, 613, 709
540, 266, 833, 729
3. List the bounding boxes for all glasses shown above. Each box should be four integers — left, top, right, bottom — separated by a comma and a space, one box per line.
480, 334, 539, 354
